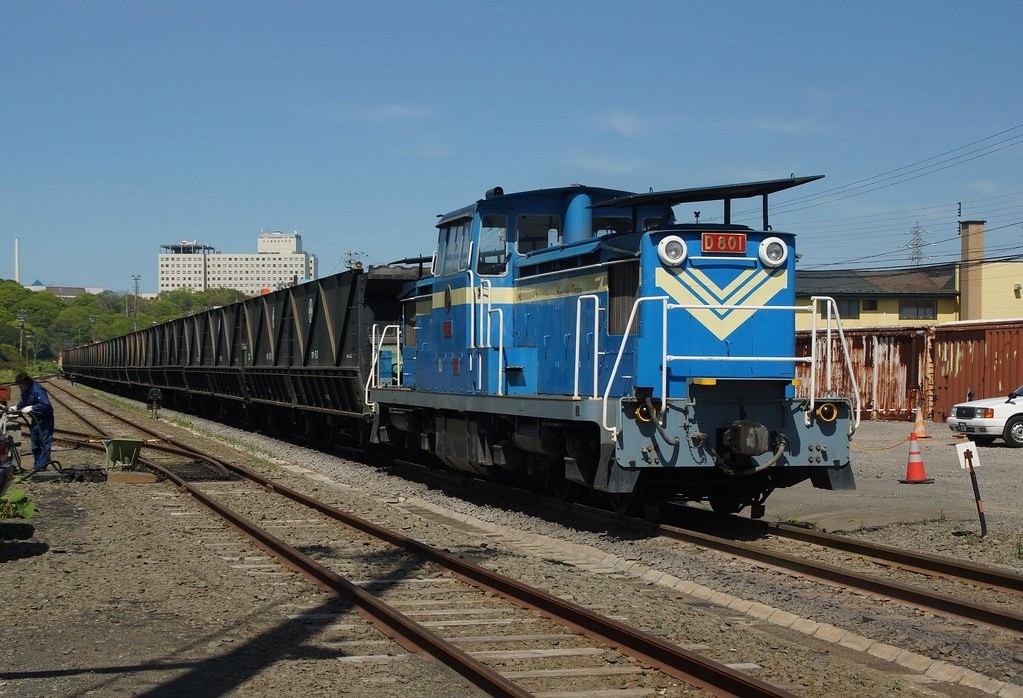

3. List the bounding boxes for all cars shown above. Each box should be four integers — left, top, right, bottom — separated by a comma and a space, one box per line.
947, 385, 1023, 448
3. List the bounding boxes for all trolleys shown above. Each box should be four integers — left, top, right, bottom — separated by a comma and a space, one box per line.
88, 439, 160, 472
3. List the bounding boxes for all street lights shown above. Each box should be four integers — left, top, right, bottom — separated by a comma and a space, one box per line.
131, 274, 141, 331
89, 313, 97, 345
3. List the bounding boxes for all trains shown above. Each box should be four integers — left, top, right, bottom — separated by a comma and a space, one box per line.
58, 174, 860, 519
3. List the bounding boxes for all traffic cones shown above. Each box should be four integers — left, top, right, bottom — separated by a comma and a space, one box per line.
897, 432, 935, 484
907, 410, 932, 441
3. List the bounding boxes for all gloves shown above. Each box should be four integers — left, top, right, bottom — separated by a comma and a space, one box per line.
8, 406, 18, 412
22, 405, 33, 414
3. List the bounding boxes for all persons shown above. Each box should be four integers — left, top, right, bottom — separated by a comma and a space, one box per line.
8, 373, 54, 473
